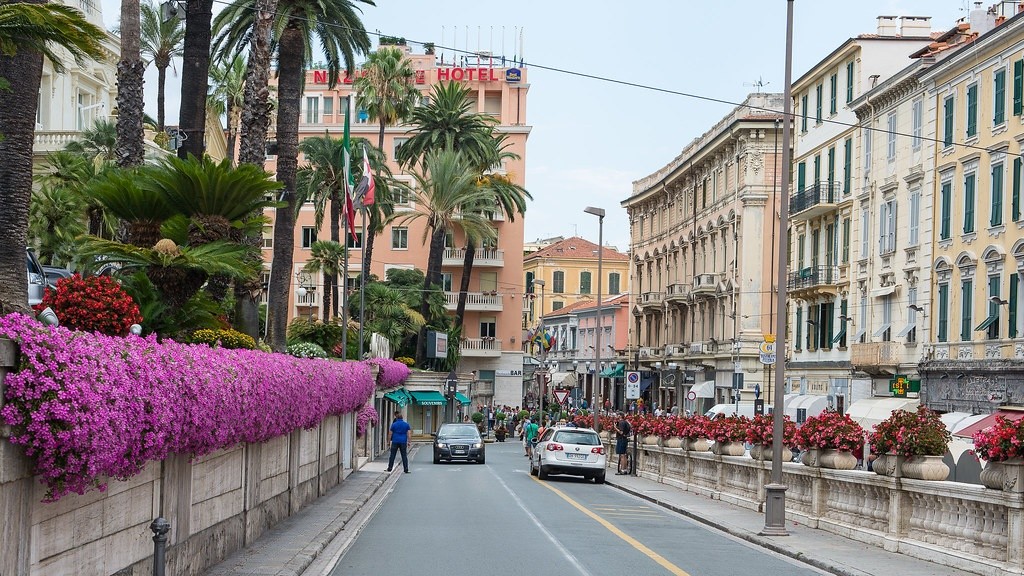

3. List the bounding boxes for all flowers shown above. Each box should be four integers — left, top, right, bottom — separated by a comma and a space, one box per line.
792, 405, 868, 450
868, 402, 953, 456
573, 413, 710, 438
709, 412, 746, 442
747, 413, 795, 445
970, 414, 1024, 462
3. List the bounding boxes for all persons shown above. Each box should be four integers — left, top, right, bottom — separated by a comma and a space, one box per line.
522, 391, 550, 412
476, 402, 536, 442
462, 415, 470, 423
629, 396, 698, 419
568, 395, 626, 417
717, 412, 725, 419
614, 413, 631, 475
384, 411, 411, 473
517, 414, 580, 460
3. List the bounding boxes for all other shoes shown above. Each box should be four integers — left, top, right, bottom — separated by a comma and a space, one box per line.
384, 469, 391, 472
615, 470, 628, 475
525, 454, 529, 456
405, 471, 411, 473
529, 457, 532, 460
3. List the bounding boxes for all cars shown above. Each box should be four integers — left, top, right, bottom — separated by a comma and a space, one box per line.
430, 421, 487, 464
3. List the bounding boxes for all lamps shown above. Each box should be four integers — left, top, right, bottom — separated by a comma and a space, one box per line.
36, 305, 60, 328
989, 295, 1008, 305
838, 315, 855, 325
587, 338, 737, 350
805, 319, 821, 328
130, 323, 142, 336
907, 305, 928, 317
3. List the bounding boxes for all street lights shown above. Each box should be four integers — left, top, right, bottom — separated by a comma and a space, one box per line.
531, 278, 546, 427
584, 206, 605, 434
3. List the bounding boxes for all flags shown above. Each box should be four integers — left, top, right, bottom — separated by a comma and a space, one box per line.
528, 324, 557, 356
342, 102, 376, 243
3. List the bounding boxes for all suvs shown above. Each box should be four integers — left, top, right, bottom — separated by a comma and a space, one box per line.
529, 425, 606, 483
26, 246, 76, 318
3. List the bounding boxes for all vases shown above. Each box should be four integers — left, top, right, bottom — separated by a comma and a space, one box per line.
750, 441, 792, 461
871, 450, 950, 480
980, 460, 1003, 489
601, 431, 745, 455
801, 444, 857, 469
711, 439, 745, 455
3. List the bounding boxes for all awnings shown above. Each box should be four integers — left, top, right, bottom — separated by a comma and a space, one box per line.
784, 393, 828, 423
843, 398, 1024, 484
383, 389, 471, 408
640, 379, 653, 391
600, 364, 624, 377
688, 379, 714, 398
